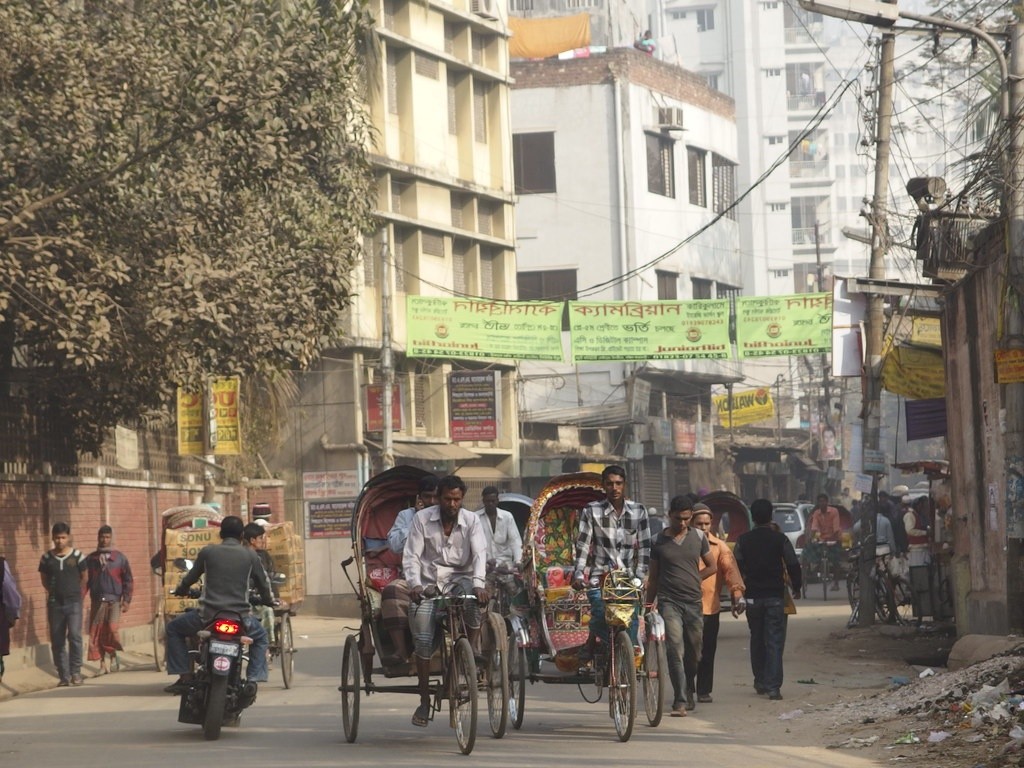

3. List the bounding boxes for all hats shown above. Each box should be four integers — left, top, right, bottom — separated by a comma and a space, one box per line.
692, 502, 713, 519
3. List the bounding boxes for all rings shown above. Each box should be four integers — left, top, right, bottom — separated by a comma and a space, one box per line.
419, 502, 421, 505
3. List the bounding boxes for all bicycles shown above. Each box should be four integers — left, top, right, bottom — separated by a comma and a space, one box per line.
846, 551, 922, 627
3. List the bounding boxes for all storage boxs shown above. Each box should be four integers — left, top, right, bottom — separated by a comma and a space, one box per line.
163, 521, 305, 616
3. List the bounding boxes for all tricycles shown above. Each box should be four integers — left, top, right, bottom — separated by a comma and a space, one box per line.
692, 489, 754, 601
152, 507, 296, 689
338, 465, 666, 754
804, 505, 855, 601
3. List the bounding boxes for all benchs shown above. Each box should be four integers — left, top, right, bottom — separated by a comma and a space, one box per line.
364, 559, 453, 675
541, 566, 648, 648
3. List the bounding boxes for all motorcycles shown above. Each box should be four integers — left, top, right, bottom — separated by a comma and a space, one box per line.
170, 558, 286, 740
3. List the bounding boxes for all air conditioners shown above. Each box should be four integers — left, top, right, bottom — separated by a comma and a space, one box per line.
658, 106, 683, 127
471, 0, 499, 21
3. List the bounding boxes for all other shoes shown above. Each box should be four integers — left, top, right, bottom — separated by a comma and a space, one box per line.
767, 689, 782, 700
164, 679, 189, 692
94, 668, 108, 678
685, 692, 695, 710
578, 647, 592, 676
57, 679, 69, 686
756, 687, 766, 696
381, 655, 408, 667
671, 704, 687, 717
72, 674, 83, 686
698, 692, 713, 702
110, 655, 119, 672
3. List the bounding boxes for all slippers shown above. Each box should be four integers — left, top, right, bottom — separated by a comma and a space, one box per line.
412, 707, 429, 727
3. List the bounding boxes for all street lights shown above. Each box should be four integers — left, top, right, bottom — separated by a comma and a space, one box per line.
801, 0, 1024, 640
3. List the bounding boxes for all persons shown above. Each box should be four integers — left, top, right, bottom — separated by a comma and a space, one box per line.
643, 488, 931, 718
820, 426, 841, 458
37, 522, 88, 687
380, 475, 523, 727
570, 466, 651, 677
242, 522, 287, 642
85, 525, 134, 678
163, 516, 274, 704
0, 556, 22, 683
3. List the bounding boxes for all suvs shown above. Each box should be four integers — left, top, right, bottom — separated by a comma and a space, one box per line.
747, 499, 815, 582
